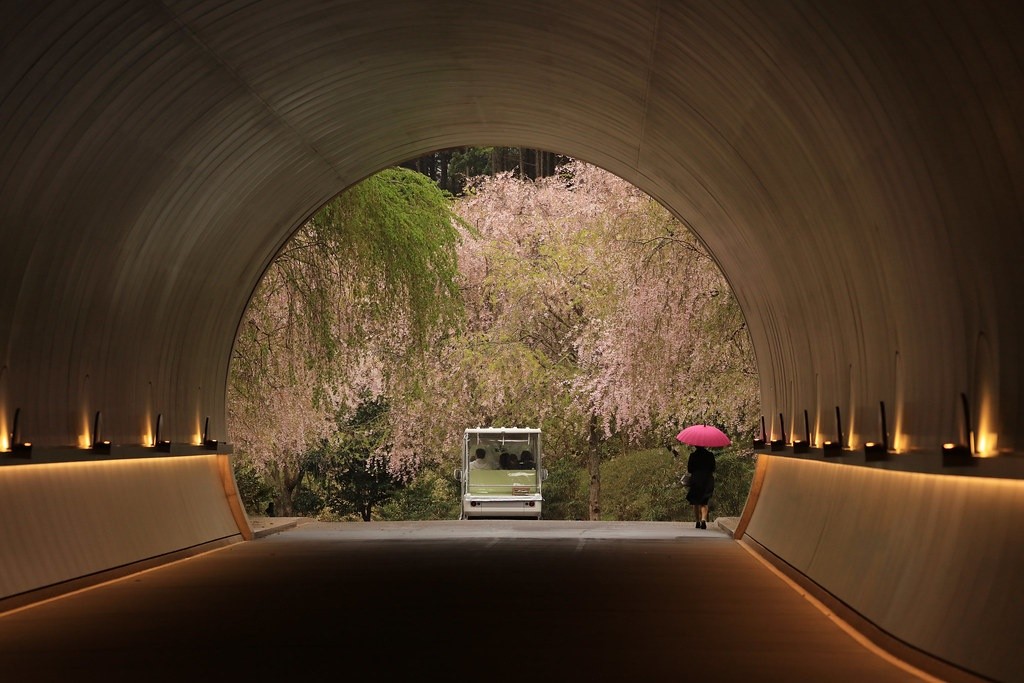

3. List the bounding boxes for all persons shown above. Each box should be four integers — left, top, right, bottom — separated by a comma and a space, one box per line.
469, 449, 492, 470
496, 452, 521, 470
521, 451, 536, 469
685, 446, 715, 529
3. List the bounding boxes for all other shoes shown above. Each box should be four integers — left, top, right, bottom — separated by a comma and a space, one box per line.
701, 520, 706, 529
696, 521, 700, 528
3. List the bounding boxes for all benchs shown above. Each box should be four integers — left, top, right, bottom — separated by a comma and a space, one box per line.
469, 469, 537, 495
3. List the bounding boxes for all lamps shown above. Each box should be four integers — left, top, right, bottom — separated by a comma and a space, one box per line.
865, 441, 884, 461
208, 439, 219, 450
793, 440, 807, 452
753, 438, 764, 450
941, 443, 971, 465
771, 439, 782, 451
160, 439, 172, 453
100, 441, 113, 453
823, 441, 839, 456
18, 443, 34, 457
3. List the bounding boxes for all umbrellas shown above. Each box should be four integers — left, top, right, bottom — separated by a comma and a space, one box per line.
676, 421, 731, 451
